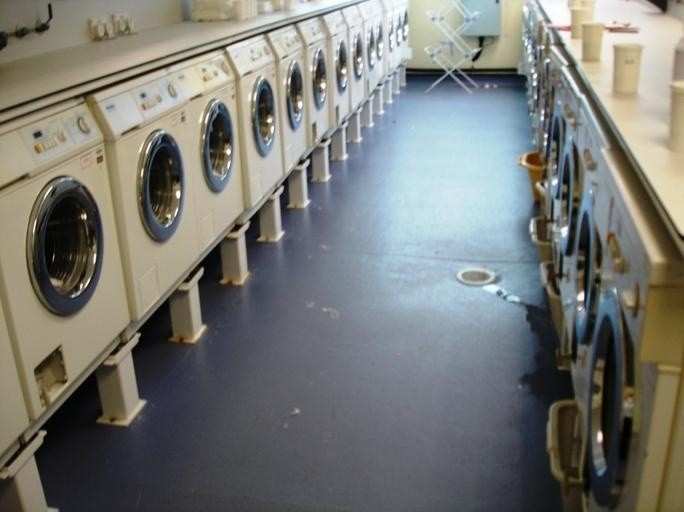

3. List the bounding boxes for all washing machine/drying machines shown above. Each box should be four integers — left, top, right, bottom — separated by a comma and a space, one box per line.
517, 0, 684, 512
0, 0, 410, 464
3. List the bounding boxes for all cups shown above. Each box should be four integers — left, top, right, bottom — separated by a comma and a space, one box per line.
570, 7, 591, 41
580, 21, 605, 64
612, 42, 644, 97
